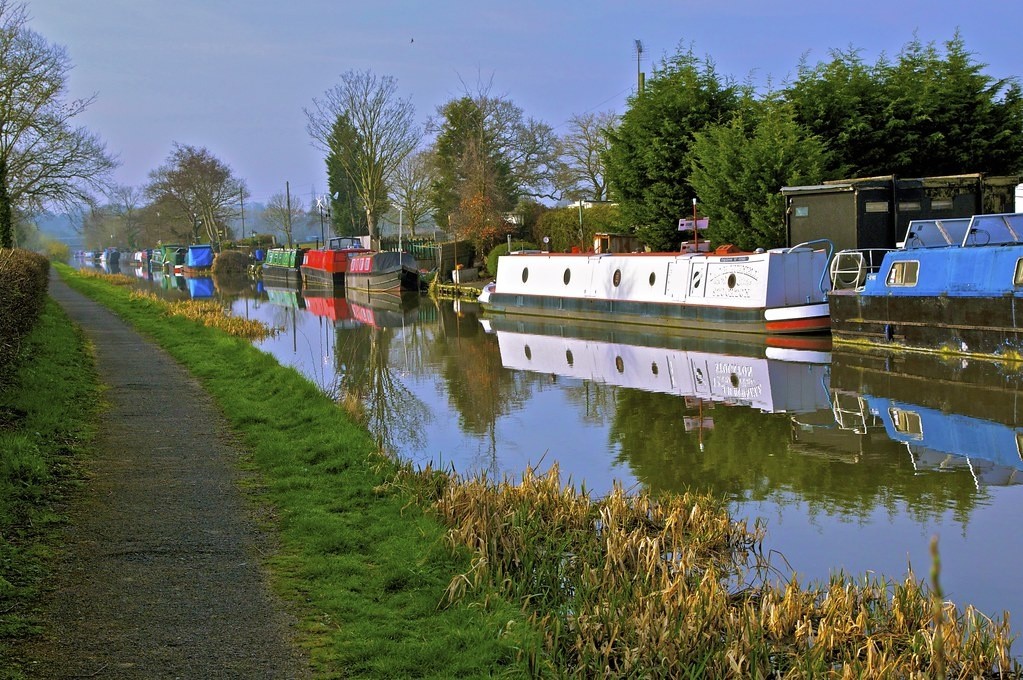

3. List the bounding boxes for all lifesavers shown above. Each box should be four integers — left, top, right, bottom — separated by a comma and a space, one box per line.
830, 249, 867, 288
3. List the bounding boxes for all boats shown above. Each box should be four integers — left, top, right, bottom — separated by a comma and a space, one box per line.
301, 281, 351, 363
82, 260, 217, 298
477, 198, 834, 334
262, 277, 306, 312
836, 336, 1023, 497
827, 212, 1023, 337
344, 210, 420, 290
345, 287, 420, 378
478, 308, 835, 454
73, 243, 213, 271
299, 236, 375, 285
262, 238, 312, 284
